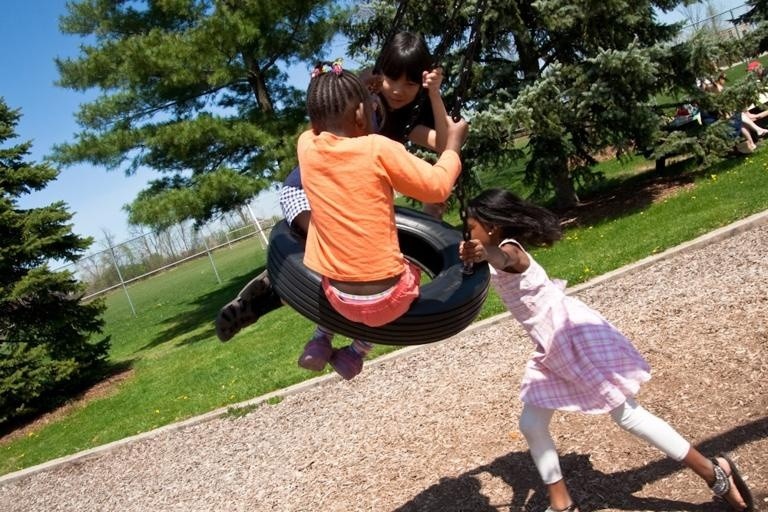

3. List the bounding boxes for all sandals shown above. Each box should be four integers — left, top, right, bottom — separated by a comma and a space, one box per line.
215, 269, 271, 343
707, 451, 756, 512
543, 498, 581, 512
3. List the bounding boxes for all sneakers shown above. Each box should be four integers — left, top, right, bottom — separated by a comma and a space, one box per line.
298, 337, 363, 381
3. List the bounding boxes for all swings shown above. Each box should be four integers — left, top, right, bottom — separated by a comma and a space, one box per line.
268, 1, 490, 345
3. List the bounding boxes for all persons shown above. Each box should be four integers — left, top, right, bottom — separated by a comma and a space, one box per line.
296, 60, 470, 383
671, 58, 768, 153
456, 187, 755, 511
215, 31, 449, 343
